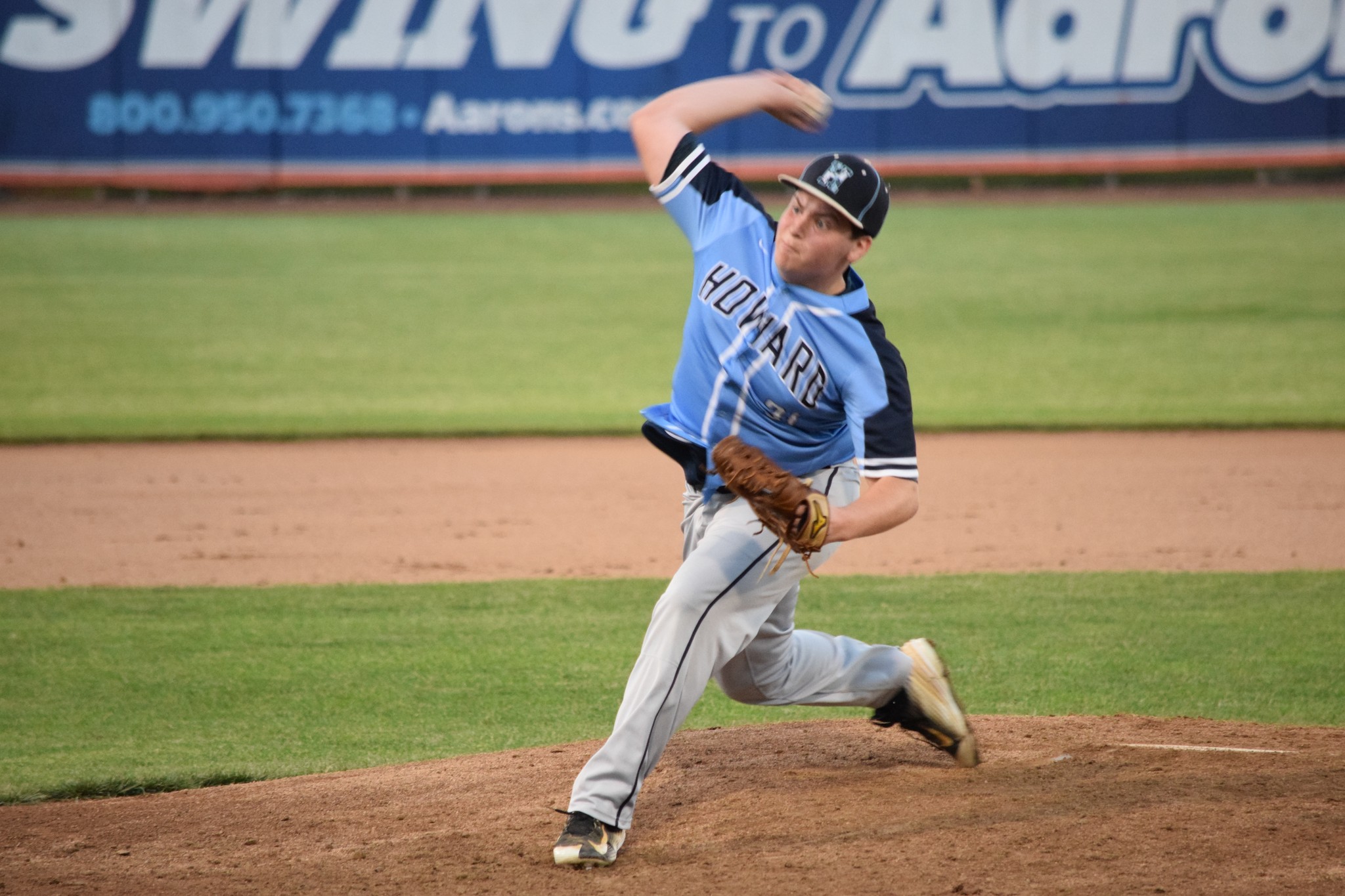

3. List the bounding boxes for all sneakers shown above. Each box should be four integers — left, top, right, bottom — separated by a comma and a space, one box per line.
547, 806, 626, 867
868, 637, 980, 769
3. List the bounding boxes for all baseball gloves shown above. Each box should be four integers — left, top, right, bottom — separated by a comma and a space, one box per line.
710, 436, 830, 553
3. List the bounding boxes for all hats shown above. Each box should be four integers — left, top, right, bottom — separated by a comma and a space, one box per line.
777, 152, 890, 238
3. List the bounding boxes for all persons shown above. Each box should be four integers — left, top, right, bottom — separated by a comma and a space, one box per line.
551, 67, 979, 869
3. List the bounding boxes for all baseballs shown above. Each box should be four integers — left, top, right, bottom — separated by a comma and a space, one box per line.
802, 84, 832, 125
